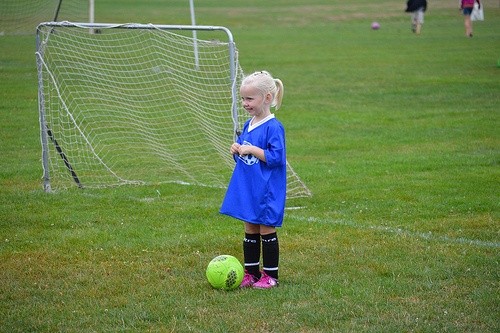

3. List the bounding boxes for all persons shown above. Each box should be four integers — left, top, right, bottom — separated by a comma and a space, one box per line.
458, 0, 481, 38
404, 0, 428, 35
218, 71, 287, 289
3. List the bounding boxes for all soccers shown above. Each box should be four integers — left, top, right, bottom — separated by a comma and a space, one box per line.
371, 22, 380, 30
206, 255, 243, 291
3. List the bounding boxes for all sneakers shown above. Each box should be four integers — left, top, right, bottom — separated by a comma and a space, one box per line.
239, 270, 263, 288
253, 270, 280, 288
